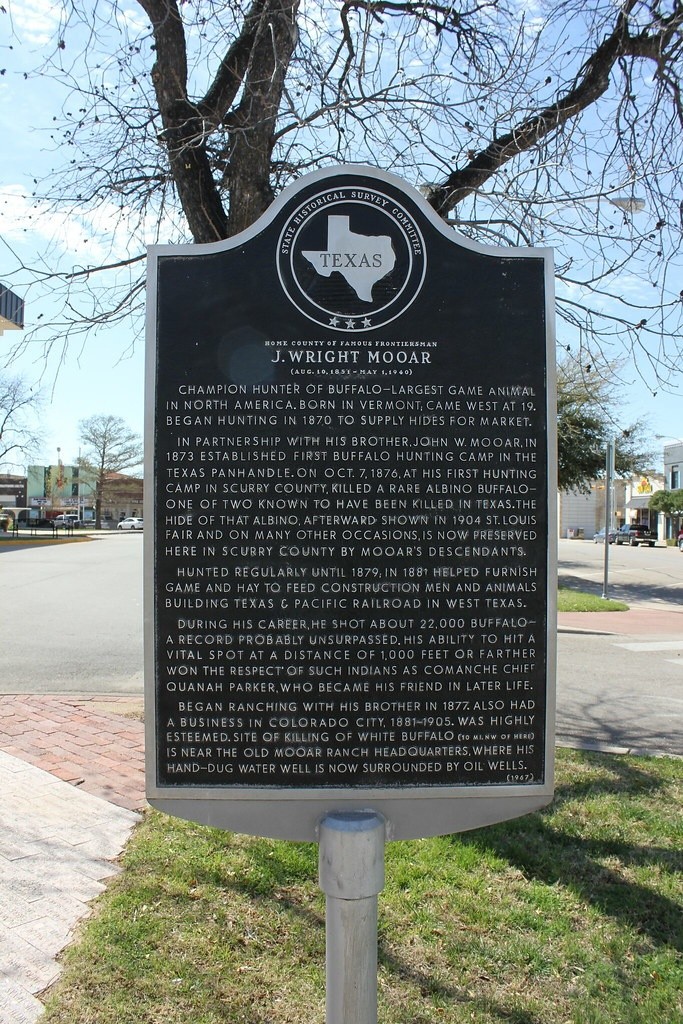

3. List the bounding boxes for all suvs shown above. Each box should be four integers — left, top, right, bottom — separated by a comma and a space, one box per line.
49, 514, 80, 530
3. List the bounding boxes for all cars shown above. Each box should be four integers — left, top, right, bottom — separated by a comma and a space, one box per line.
117, 517, 143, 530
593, 526, 617, 544
677, 529, 683, 553
91, 514, 118, 526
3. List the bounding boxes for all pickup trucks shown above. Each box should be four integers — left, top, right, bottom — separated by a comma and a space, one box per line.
612, 524, 659, 547
